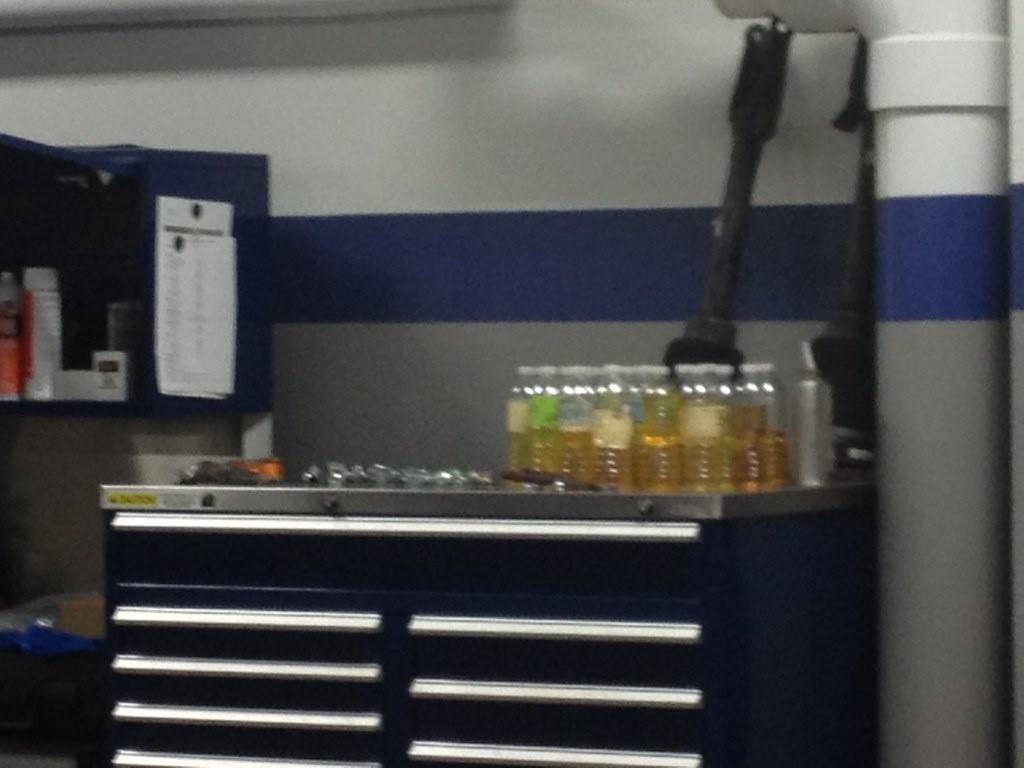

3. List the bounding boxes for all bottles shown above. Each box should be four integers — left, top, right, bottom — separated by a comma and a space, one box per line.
505, 360, 789, 492
0, 267, 62, 401
790, 368, 831, 487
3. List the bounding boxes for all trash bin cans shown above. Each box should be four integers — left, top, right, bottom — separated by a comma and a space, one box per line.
0, 591, 107, 768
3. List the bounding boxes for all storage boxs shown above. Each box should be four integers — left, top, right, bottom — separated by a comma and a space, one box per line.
57, 597, 110, 638
